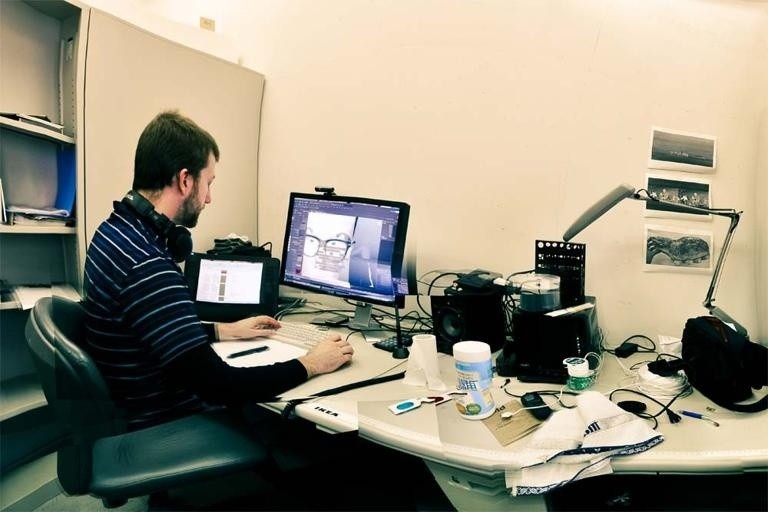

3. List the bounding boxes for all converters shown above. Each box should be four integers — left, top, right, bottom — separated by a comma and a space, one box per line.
615, 343, 638, 358
647, 358, 672, 378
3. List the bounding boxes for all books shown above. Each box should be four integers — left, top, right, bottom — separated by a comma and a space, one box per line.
0, 111, 66, 135
0, 176, 78, 228
1, 276, 84, 313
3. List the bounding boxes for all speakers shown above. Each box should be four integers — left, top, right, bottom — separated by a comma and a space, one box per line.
430, 295, 508, 358
512, 296, 600, 385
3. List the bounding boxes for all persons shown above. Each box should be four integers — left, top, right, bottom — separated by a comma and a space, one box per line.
653, 184, 709, 210
77, 108, 357, 455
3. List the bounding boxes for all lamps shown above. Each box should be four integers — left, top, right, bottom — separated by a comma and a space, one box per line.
559, 182, 742, 361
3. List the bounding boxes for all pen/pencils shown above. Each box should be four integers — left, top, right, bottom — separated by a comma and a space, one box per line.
227, 346, 270, 359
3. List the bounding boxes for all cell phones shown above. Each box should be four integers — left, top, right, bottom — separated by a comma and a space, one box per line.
522, 392, 552, 420
326, 315, 349, 325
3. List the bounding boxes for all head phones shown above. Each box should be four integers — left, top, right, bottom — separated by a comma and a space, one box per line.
122, 191, 192, 263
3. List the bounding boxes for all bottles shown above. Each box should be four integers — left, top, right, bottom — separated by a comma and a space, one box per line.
452, 339, 497, 421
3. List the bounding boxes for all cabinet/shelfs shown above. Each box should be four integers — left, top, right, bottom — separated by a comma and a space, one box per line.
0, 2, 268, 511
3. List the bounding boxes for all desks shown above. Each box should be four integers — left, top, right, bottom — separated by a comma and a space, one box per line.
206, 291, 767, 512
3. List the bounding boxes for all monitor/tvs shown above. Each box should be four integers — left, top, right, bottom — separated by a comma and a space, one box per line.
183, 251, 280, 322
277, 192, 411, 343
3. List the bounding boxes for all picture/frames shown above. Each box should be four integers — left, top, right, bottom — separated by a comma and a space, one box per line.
642, 127, 720, 270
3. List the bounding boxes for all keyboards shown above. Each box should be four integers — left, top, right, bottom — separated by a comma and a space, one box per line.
260, 321, 338, 352
